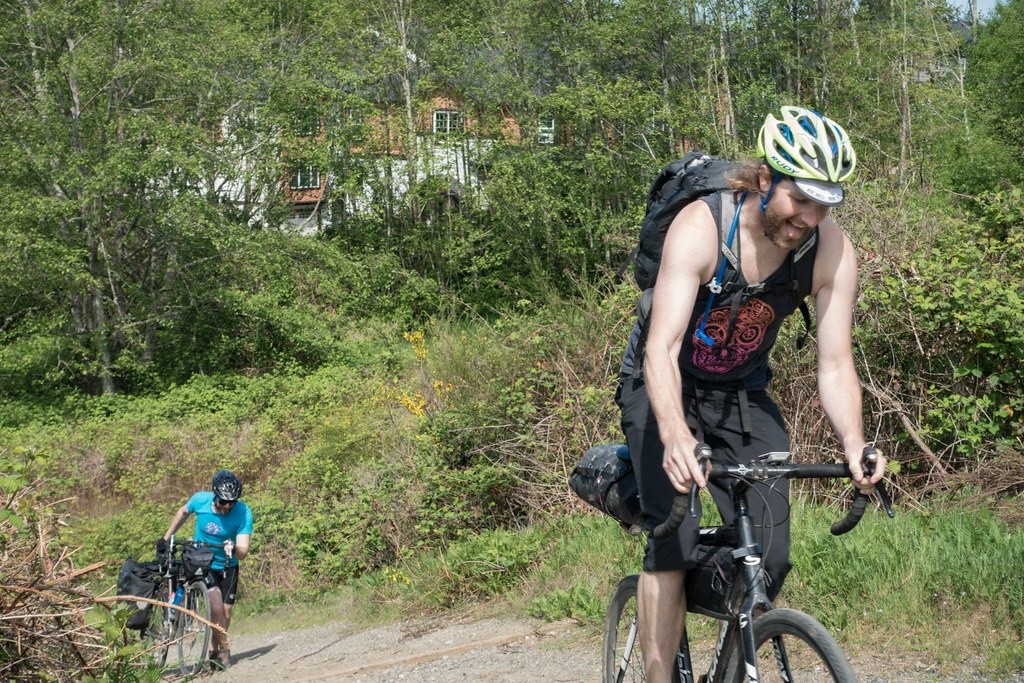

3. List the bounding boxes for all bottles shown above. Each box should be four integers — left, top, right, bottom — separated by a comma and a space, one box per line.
170, 588, 184, 615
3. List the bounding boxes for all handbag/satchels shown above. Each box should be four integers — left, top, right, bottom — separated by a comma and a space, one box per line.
116, 557, 164, 630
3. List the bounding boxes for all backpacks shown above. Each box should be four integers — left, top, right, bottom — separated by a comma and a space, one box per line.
618, 152, 810, 379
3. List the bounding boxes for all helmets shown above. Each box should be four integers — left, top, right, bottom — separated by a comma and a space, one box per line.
755, 105, 856, 206
212, 470, 242, 500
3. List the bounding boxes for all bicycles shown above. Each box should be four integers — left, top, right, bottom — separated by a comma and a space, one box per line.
601, 442, 896, 683
140, 534, 234, 677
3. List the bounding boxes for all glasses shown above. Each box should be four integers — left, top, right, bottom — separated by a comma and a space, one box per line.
216, 496, 236, 505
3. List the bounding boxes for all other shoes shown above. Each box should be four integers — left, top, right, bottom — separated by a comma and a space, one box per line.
209, 647, 231, 671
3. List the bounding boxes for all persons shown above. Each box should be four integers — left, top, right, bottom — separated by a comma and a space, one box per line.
154, 470, 253, 670
615, 106, 885, 683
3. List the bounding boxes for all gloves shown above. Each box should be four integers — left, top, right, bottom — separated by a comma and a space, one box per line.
156, 538, 167, 554
